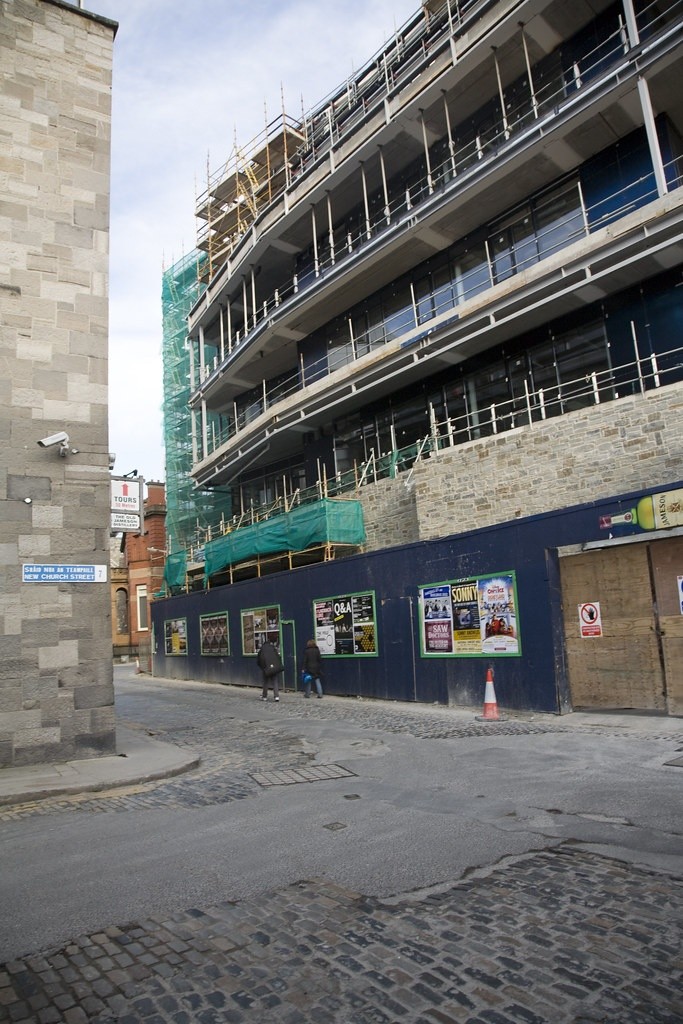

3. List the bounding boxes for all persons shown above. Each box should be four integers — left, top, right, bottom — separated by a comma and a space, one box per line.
257, 642, 280, 701
425, 599, 450, 617
301, 639, 323, 698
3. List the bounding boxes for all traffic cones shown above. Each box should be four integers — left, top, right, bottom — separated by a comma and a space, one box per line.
134, 656, 141, 675
475, 669, 508, 722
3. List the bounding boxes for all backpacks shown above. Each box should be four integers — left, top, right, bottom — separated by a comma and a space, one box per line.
261, 643, 285, 677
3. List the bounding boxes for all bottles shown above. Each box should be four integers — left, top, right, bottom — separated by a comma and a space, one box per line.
599, 488, 683, 531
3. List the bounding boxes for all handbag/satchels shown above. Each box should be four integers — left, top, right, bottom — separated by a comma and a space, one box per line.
300, 671, 313, 684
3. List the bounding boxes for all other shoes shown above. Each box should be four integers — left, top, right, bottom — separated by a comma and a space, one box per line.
304, 694, 310, 698
274, 696, 280, 700
317, 695, 323, 698
260, 696, 267, 700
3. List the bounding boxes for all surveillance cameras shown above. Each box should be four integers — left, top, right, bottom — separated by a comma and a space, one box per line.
37, 431, 69, 449
